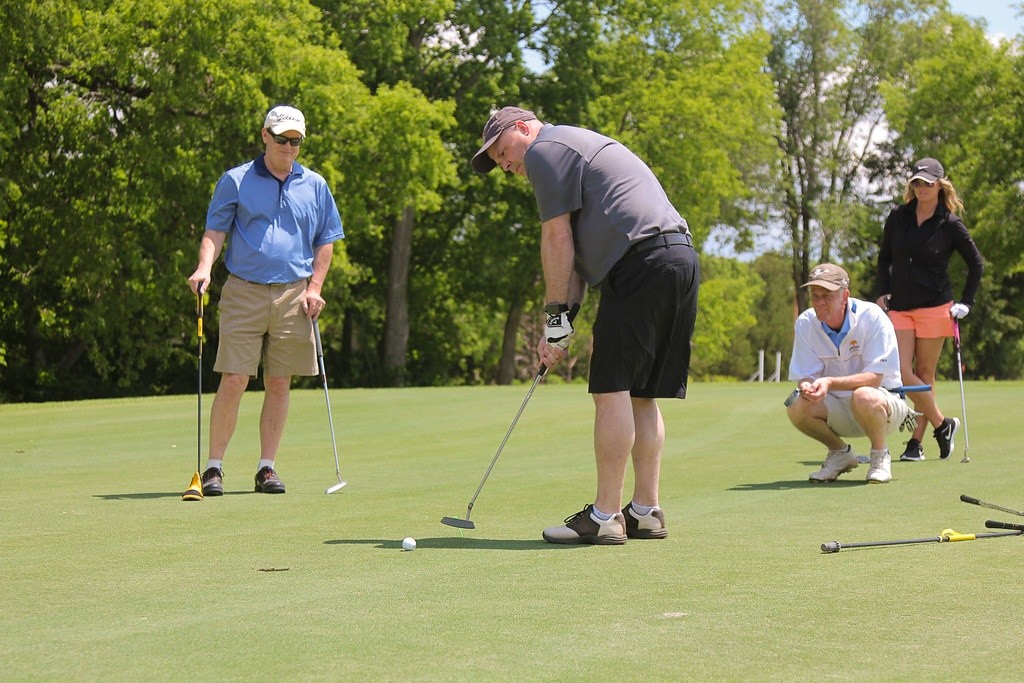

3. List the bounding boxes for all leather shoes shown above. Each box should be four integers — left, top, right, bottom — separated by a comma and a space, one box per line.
201, 467, 225, 496
254, 466, 285, 493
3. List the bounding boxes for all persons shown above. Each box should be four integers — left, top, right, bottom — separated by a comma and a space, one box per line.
188, 105, 345, 495
470, 106, 698, 546
874, 157, 983, 461
783, 263, 910, 484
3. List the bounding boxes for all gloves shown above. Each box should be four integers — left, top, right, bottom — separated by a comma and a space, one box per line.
544, 303, 574, 354
950, 303, 969, 319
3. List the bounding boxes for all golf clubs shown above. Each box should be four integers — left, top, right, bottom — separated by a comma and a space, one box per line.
440, 302, 581, 530
784, 384, 932, 407
960, 494, 1024, 516
985, 520, 1024, 530
182, 281, 204, 502
953, 315, 970, 463
313, 320, 347, 494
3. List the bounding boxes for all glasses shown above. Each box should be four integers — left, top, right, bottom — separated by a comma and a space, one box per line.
267, 128, 303, 146
913, 180, 935, 188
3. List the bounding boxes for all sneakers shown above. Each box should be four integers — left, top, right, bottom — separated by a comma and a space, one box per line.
622, 501, 668, 539
866, 450, 892, 484
809, 445, 858, 483
899, 439, 925, 461
933, 417, 961, 459
543, 505, 628, 544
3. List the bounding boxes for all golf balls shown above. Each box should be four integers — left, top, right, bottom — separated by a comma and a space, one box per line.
402, 537, 417, 551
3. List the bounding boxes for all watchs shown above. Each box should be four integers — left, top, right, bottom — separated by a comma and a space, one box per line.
544, 303, 569, 313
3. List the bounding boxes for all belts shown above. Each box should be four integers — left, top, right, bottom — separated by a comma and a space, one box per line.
617, 234, 693, 263
230, 273, 305, 287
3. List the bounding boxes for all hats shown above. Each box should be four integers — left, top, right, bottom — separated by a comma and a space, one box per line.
799, 264, 849, 291
910, 158, 944, 183
263, 105, 307, 140
472, 106, 537, 173
